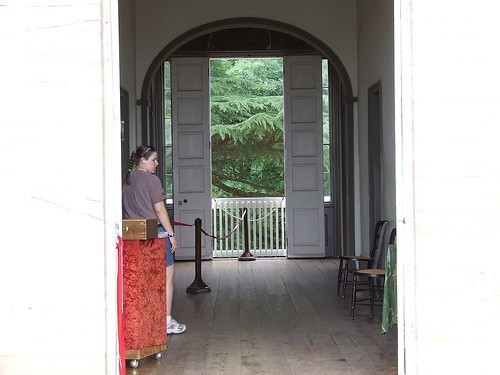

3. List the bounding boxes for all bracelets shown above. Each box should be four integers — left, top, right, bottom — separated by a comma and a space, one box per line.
167, 233, 176, 238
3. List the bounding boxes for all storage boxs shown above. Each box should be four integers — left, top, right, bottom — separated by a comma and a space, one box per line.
122, 218, 158, 240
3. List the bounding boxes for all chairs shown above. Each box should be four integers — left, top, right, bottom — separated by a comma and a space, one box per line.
336, 220, 389, 300
350, 228, 396, 320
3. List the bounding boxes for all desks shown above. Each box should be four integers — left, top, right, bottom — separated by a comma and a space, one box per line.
123, 237, 168, 368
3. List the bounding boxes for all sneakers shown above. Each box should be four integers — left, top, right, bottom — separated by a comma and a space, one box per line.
167, 319, 187, 334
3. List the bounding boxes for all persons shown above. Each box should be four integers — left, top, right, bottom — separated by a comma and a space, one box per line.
122, 145, 187, 335
